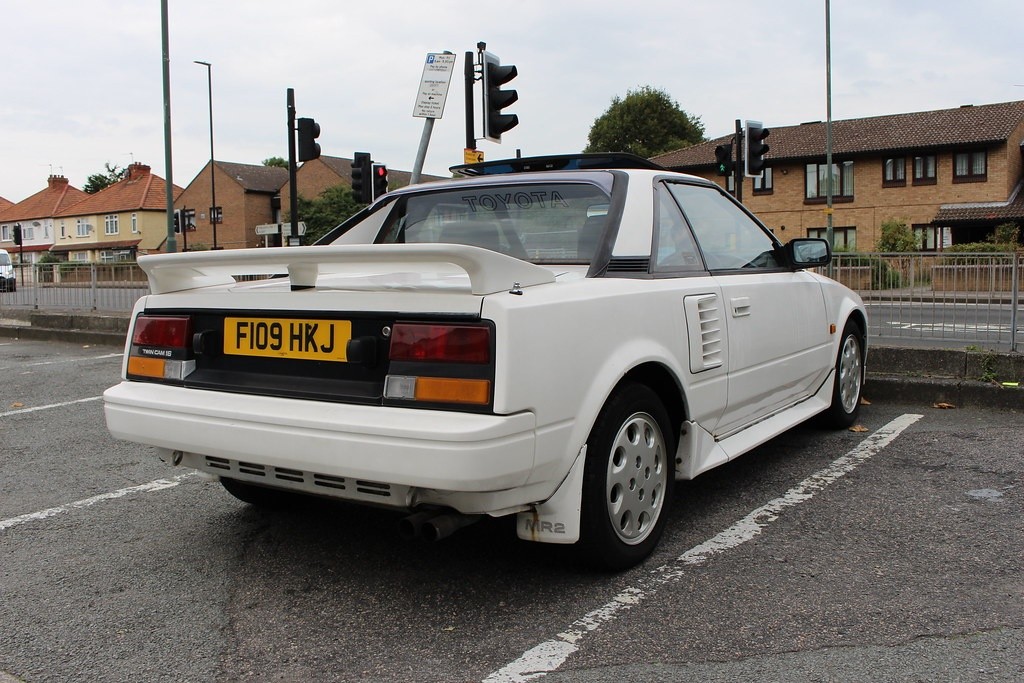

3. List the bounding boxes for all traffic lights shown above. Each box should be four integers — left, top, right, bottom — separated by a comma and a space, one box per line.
350, 152, 371, 204
297, 118, 321, 163
745, 120, 771, 178
372, 164, 390, 202
173, 209, 181, 234
480, 49, 520, 145
714, 144, 732, 178
12, 225, 21, 245
194, 61, 217, 248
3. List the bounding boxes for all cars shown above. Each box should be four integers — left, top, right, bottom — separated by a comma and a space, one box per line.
0, 249, 16, 292
102, 149, 870, 573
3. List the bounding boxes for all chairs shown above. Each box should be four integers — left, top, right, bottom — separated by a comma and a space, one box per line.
437, 220, 501, 253
576, 214, 611, 259
